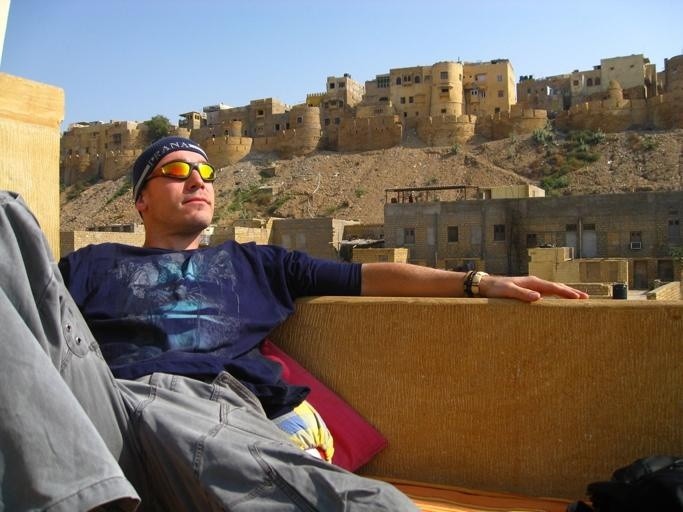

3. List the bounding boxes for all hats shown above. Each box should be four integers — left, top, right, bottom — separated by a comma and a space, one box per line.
132, 136, 209, 202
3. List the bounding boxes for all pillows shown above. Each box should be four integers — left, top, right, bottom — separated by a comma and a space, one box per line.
254, 338, 392, 483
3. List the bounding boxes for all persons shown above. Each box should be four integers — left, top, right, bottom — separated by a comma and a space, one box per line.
0, 134, 587, 512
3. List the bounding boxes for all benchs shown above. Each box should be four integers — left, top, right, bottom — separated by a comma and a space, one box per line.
269, 295, 681, 512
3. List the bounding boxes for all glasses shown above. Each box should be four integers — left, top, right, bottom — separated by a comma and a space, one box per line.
146, 158, 216, 182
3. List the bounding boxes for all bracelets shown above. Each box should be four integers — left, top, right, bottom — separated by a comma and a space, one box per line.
462, 270, 489, 296
